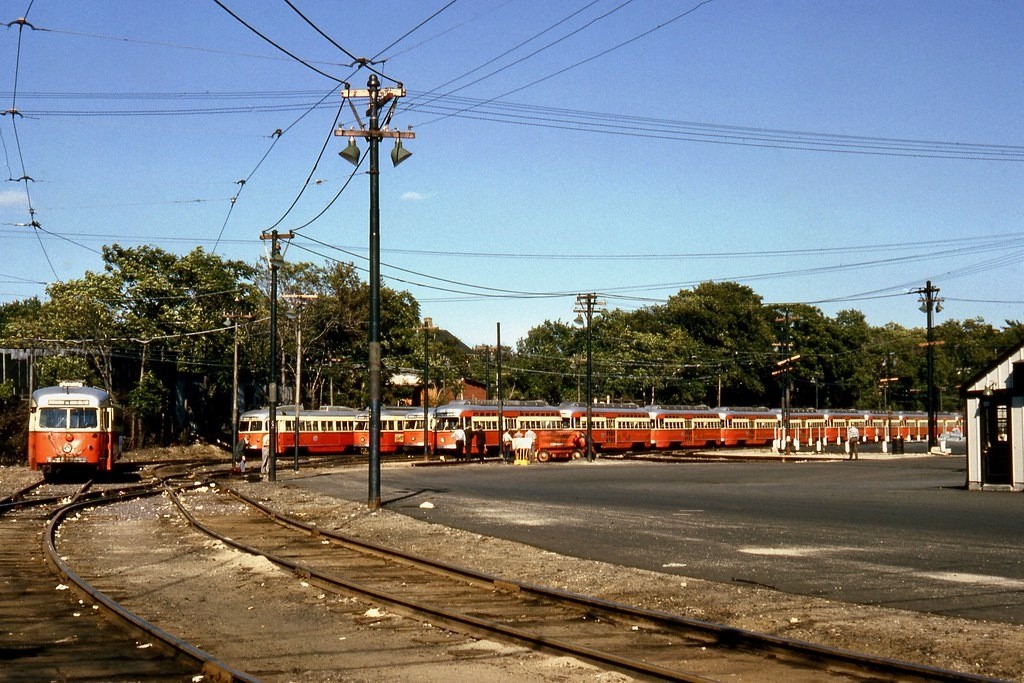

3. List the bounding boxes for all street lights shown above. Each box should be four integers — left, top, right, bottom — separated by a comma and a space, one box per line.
333, 74, 417, 507
573, 292, 606, 461
259, 229, 296, 482
915, 280, 944, 452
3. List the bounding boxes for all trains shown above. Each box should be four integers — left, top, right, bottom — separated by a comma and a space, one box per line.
354, 406, 411, 455
401, 409, 437, 455
433, 397, 966, 457
26, 378, 124, 476
239, 404, 359, 456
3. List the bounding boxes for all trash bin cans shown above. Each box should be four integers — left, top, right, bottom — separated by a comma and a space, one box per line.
891, 435, 905, 456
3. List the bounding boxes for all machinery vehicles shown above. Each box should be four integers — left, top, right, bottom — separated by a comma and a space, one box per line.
537, 429, 596, 462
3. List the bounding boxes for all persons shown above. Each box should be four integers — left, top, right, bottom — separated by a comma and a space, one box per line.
261, 434, 270, 475
451, 425, 466, 462
513, 428, 524, 438
525, 426, 537, 464
848, 422, 860, 460
502, 429, 513, 460
464, 426, 474, 462
475, 429, 487, 460
235, 434, 250, 472
